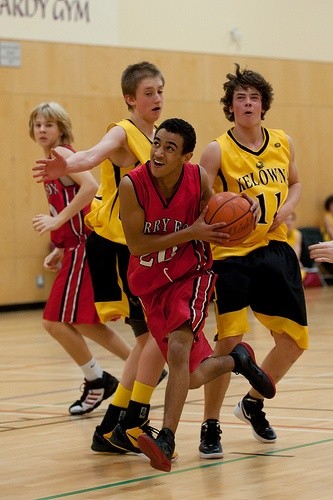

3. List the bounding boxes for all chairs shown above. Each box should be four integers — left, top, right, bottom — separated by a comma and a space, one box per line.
294, 225, 333, 289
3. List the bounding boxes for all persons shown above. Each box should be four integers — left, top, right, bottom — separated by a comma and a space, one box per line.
319, 193, 333, 286
285, 211, 307, 289
307, 238, 333, 263
28, 102, 169, 416
116, 118, 277, 473
198, 61, 312, 460
31, 62, 170, 456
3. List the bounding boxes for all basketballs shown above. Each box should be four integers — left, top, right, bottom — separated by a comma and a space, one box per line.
206, 192, 254, 247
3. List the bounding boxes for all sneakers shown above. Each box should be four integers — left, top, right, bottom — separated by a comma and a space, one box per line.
229, 342, 277, 399
155, 370, 168, 385
137, 427, 176, 472
91, 425, 134, 453
233, 397, 278, 443
109, 419, 178, 460
69, 370, 120, 415
198, 418, 225, 459
